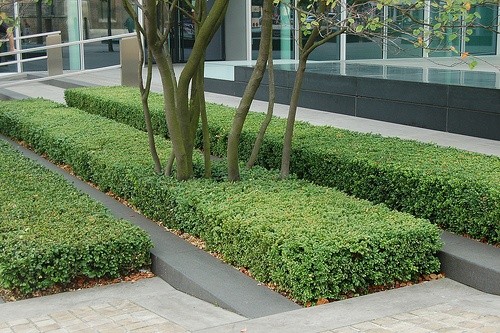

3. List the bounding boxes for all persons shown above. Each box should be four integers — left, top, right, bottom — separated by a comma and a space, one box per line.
126, 16, 135, 33
0, 21, 15, 52
